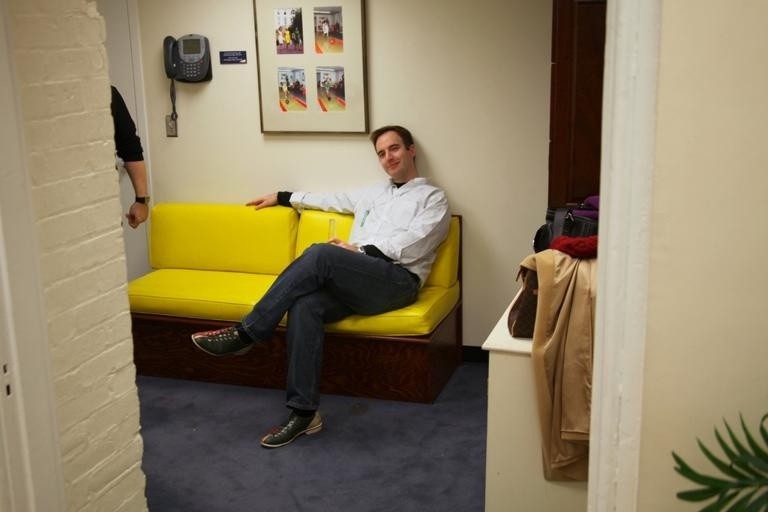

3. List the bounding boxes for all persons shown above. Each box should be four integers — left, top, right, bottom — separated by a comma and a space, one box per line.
192, 126, 451, 448
320, 72, 332, 100
111, 85, 148, 229
322, 20, 339, 37
276, 26, 302, 52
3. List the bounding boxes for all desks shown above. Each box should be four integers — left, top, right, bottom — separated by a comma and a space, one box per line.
479, 284, 588, 512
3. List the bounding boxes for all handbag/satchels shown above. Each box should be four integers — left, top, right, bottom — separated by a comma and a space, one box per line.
507, 267, 538, 338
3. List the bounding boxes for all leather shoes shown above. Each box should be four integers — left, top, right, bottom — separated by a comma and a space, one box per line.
259, 410, 323, 448
191, 326, 256, 357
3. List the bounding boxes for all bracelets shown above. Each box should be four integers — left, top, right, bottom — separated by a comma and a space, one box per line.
357, 246, 366, 254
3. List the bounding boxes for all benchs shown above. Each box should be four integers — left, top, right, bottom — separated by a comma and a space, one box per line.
126, 200, 466, 406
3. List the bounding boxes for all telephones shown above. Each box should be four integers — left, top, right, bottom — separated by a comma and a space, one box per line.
164, 34, 212, 83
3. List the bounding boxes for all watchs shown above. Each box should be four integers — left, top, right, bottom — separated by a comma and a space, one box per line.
135, 196, 149, 204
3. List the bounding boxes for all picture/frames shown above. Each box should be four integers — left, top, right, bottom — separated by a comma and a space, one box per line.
252, 0, 369, 136
